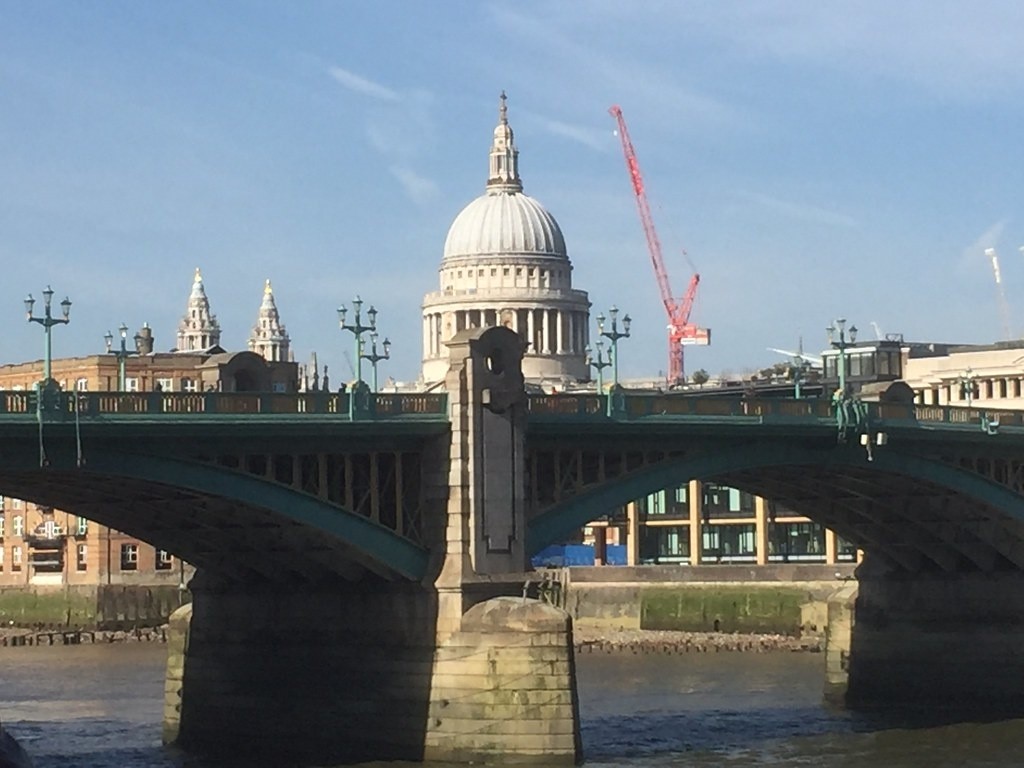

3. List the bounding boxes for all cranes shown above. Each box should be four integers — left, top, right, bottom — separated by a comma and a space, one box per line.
608, 103, 710, 392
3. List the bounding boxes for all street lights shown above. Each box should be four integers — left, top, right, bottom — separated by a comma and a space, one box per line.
597, 305, 632, 394
959, 364, 980, 408
103, 324, 143, 391
337, 295, 378, 422
358, 331, 391, 394
824, 318, 860, 399
23, 284, 73, 389
583, 341, 613, 396
